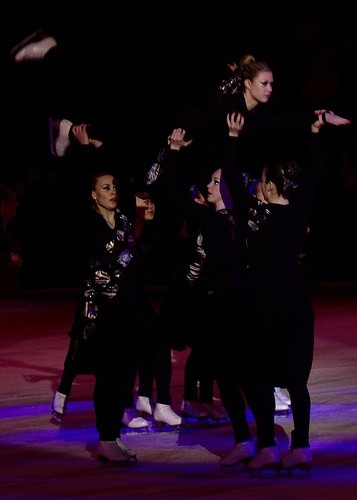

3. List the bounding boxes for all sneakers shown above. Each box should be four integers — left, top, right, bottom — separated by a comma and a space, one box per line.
96, 441, 130, 465
136, 396, 152, 417
116, 438, 137, 457
10, 33, 57, 63
121, 411, 149, 435
49, 118, 73, 158
50, 390, 67, 421
154, 403, 181, 426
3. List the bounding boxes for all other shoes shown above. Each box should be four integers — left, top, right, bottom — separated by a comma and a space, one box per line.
181, 400, 209, 417
246, 445, 281, 471
274, 392, 289, 418
218, 439, 255, 468
282, 447, 314, 470
202, 403, 226, 419
275, 387, 291, 406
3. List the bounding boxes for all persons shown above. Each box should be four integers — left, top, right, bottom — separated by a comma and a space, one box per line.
10, 25, 350, 477
51, 123, 137, 430
